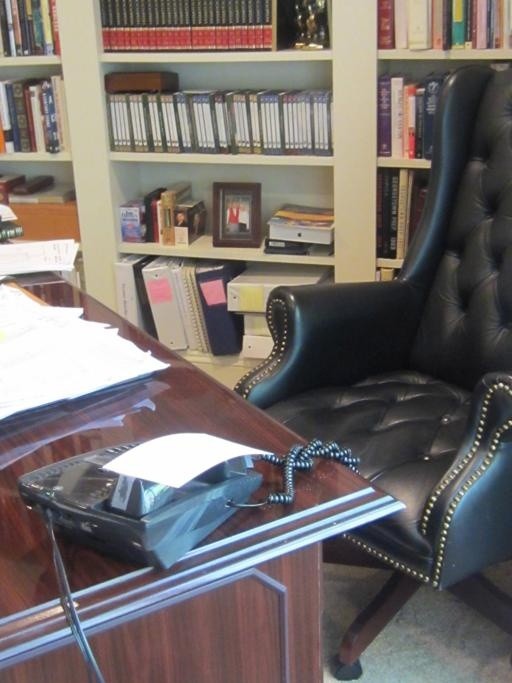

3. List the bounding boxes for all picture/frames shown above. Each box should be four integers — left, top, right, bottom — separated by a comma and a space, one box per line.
210, 179, 263, 249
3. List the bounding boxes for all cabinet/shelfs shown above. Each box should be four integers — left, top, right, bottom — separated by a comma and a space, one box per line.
340, 0, 511, 284
0, 0, 88, 261
93, 1, 338, 374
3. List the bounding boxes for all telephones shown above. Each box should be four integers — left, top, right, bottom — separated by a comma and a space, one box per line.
16, 440, 263, 570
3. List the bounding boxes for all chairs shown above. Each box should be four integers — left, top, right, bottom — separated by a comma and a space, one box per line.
233, 59, 510, 679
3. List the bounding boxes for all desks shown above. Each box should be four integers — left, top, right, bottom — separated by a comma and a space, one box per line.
0, 240, 409, 680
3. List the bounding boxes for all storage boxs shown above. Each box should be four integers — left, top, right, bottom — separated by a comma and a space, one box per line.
7, 200, 80, 243
